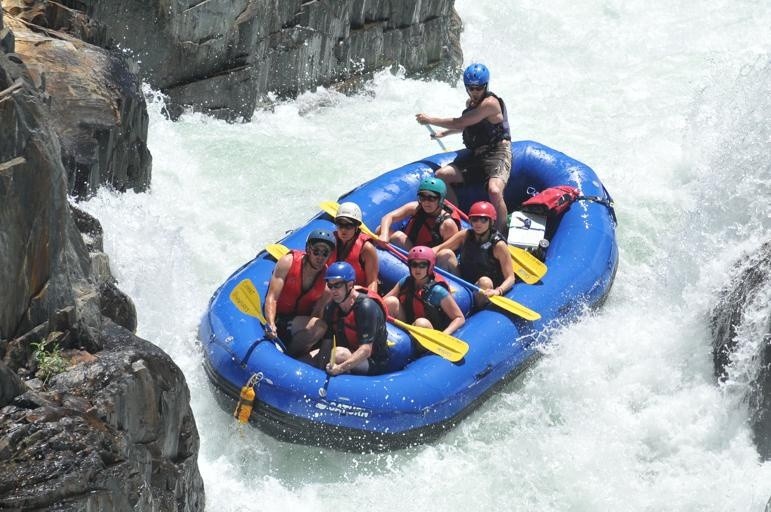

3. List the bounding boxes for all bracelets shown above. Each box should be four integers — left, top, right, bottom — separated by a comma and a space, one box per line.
497, 286, 504, 296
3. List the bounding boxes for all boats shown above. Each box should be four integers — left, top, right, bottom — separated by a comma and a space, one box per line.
190, 137, 626, 455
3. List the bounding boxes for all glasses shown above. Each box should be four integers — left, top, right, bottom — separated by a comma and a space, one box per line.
335, 223, 354, 230
327, 281, 343, 290
312, 249, 330, 257
409, 261, 428, 268
470, 217, 488, 223
467, 85, 485, 92
419, 195, 438, 202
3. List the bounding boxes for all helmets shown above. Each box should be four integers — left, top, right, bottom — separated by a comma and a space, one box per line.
463, 63, 490, 91
417, 177, 446, 207
467, 201, 496, 221
334, 202, 361, 226
407, 246, 436, 275
324, 262, 355, 283
305, 230, 336, 252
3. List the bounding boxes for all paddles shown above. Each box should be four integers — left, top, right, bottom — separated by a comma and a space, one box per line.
230, 278, 291, 355
386, 239, 542, 322
264, 242, 290, 261
328, 334, 338, 371
442, 196, 549, 285
384, 312, 470, 363
319, 200, 409, 265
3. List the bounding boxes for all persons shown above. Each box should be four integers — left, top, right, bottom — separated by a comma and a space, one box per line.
324, 201, 380, 297
383, 245, 465, 354
415, 62, 513, 234
431, 200, 516, 308
375, 177, 462, 258
263, 228, 337, 365
316, 261, 388, 378
